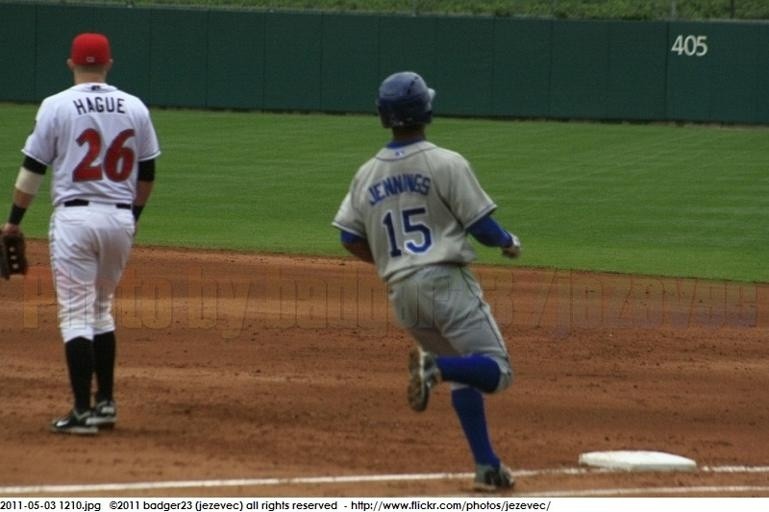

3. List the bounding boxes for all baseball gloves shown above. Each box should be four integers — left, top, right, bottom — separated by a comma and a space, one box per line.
1, 229, 27, 279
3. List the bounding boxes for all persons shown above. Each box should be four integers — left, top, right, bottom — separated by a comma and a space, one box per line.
0, 33, 162, 436
332, 70, 522, 492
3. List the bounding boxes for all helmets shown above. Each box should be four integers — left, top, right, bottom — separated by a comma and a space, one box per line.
375, 71, 437, 128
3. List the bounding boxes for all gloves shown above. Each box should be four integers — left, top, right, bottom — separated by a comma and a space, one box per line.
501, 232, 522, 259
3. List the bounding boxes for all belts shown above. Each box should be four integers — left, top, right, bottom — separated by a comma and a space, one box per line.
64, 199, 133, 210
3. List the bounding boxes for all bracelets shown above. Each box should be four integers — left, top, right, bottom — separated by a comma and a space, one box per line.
8, 204, 26, 226
132, 204, 144, 224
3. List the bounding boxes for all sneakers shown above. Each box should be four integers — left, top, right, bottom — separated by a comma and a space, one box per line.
94, 401, 117, 427
470, 459, 516, 492
48, 407, 98, 435
406, 343, 443, 411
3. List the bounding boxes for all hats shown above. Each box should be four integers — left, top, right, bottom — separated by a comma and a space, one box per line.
69, 33, 111, 67
